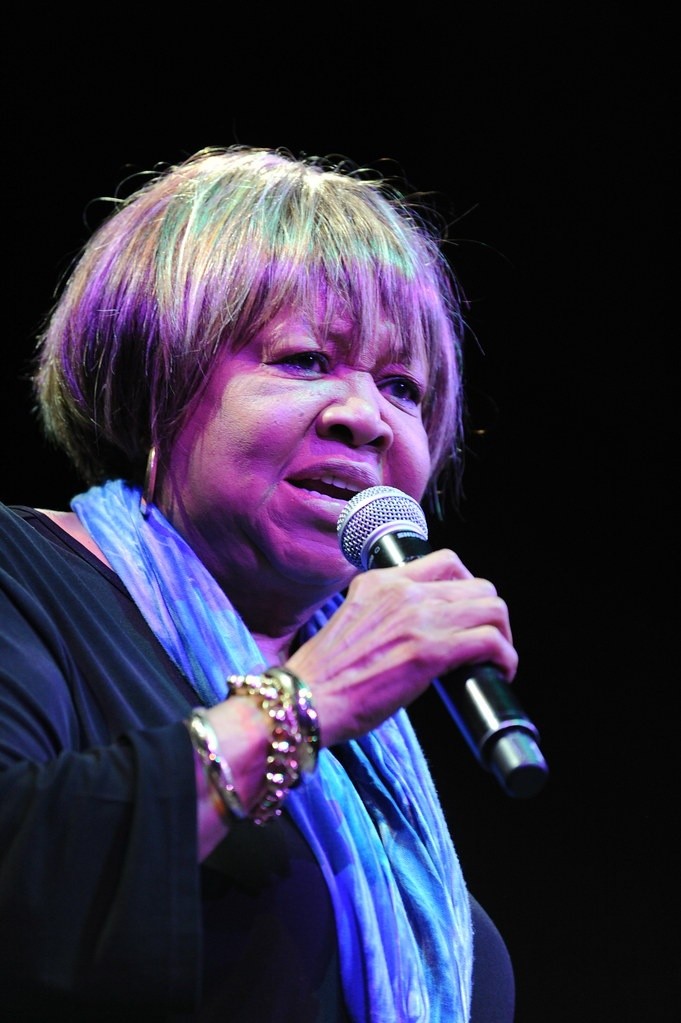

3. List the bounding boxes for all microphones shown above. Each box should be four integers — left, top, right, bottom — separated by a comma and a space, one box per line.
337, 485, 549, 798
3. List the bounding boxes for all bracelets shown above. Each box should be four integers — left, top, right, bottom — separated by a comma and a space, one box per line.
188, 665, 321, 824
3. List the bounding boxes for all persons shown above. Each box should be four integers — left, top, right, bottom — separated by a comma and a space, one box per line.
0, 151, 518, 1023
